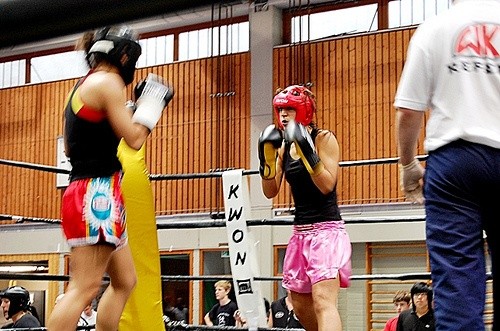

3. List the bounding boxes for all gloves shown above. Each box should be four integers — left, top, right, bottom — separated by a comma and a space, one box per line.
257, 124, 283, 180
283, 119, 324, 177
133, 72, 176, 134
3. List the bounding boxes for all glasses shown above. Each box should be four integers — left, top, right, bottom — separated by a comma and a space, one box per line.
413, 292, 427, 297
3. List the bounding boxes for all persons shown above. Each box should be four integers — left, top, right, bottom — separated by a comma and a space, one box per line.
382, 290, 411, 331
234, 297, 270, 327
46, 25, 176, 331
0, 285, 41, 331
163, 295, 188, 324
55, 294, 87, 331
266, 286, 304, 328
80, 303, 97, 331
258, 85, 352, 330
396, 282, 435, 331
393, 0, 500, 331
204, 281, 237, 326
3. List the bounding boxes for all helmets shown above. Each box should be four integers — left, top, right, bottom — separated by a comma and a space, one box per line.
87, 22, 142, 85
274, 82, 315, 129
0, 286, 30, 320
